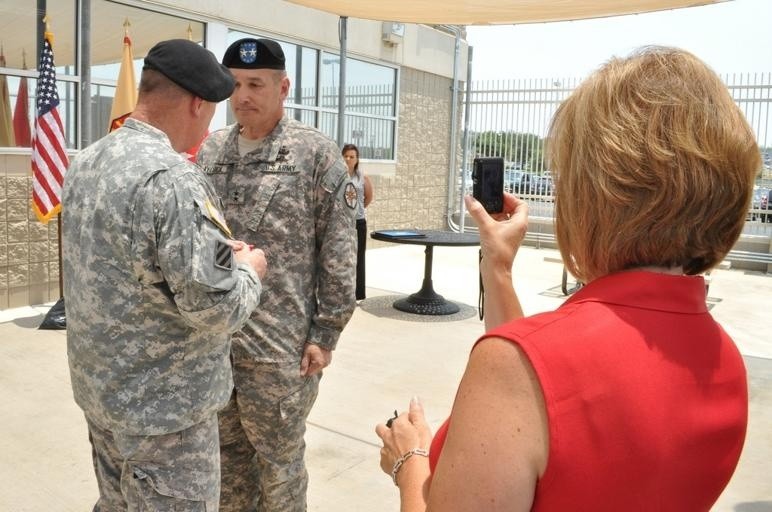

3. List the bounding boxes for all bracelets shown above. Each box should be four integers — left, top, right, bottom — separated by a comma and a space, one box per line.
391, 448, 428, 488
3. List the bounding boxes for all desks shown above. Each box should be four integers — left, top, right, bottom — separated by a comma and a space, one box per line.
370, 229, 481, 315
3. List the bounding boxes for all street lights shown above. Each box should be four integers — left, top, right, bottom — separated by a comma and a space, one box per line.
322, 56, 341, 143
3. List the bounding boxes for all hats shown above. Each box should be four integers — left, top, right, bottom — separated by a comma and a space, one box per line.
219, 37, 287, 71
140, 39, 236, 103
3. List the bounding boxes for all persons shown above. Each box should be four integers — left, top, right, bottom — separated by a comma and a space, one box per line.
193, 38, 354, 512
341, 141, 374, 304
375, 44, 762, 512
63, 36, 268, 511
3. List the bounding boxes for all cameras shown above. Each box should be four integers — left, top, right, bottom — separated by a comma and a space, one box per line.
471, 156, 506, 215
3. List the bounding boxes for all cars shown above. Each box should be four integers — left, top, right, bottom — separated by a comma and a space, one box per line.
747, 188, 772, 223
456, 155, 559, 195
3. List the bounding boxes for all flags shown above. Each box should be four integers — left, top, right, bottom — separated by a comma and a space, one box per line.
0, 52, 16, 145
12, 68, 31, 145
105, 37, 138, 132
181, 34, 213, 163
31, 23, 69, 225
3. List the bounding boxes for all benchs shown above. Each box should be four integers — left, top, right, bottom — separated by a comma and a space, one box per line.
543, 256, 731, 298
